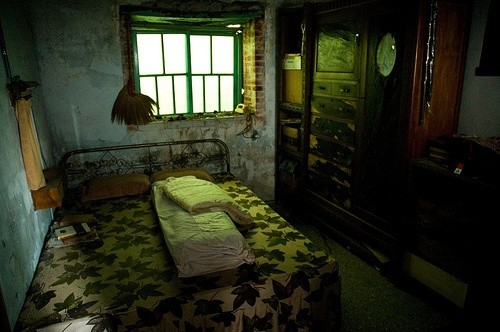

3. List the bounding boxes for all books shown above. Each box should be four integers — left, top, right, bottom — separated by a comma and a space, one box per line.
53, 214, 97, 244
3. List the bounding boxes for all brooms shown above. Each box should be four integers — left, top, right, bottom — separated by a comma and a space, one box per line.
111, 14, 160, 125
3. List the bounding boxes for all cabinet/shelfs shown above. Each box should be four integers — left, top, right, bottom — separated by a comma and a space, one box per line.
398, 156, 500, 332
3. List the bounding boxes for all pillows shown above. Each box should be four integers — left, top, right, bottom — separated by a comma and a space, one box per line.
81, 173, 150, 202
151, 168, 216, 184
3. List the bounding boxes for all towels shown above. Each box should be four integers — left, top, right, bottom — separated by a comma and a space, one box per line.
15, 97, 47, 191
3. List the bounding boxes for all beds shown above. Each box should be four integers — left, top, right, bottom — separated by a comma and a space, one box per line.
14, 138, 343, 332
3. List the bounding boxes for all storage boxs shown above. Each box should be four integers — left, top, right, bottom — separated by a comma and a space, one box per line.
272, 1, 468, 287
281, 53, 303, 104
284, 126, 300, 151
280, 157, 298, 190
